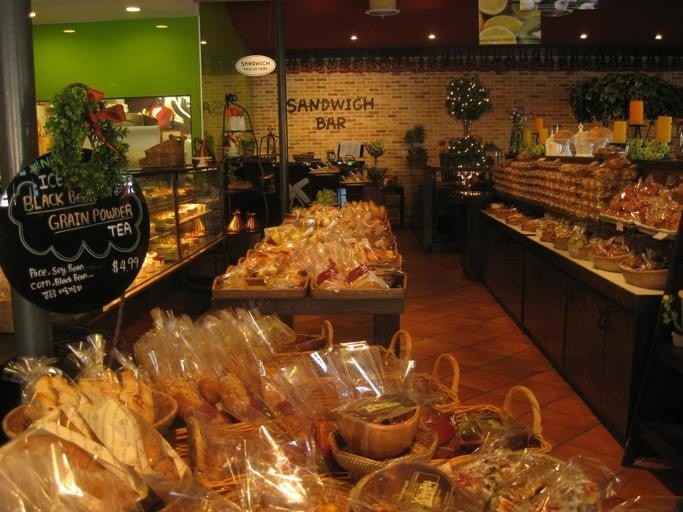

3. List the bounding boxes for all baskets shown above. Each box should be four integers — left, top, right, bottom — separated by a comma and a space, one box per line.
618, 260, 668, 290
284, 353, 461, 415
329, 424, 439, 472
440, 450, 602, 511
590, 249, 631, 273
431, 385, 553, 467
171, 395, 283, 459
153, 407, 396, 512
265, 329, 412, 377
273, 319, 333, 367
2, 387, 178, 440
347, 460, 483, 512
552, 236, 571, 250
567, 240, 592, 260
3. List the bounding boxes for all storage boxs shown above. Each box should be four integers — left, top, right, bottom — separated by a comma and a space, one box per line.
212, 272, 310, 300
309, 268, 408, 299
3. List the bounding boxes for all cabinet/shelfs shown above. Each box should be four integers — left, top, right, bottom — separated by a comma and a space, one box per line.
490, 153, 683, 253
474, 212, 524, 327
565, 280, 640, 440
523, 235, 566, 373
101, 164, 227, 321
211, 298, 404, 358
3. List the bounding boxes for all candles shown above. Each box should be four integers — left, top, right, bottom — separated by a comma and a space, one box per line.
627, 97, 649, 136
524, 131, 531, 145
658, 116, 673, 159
537, 115, 544, 133
610, 120, 626, 145
538, 128, 546, 143
